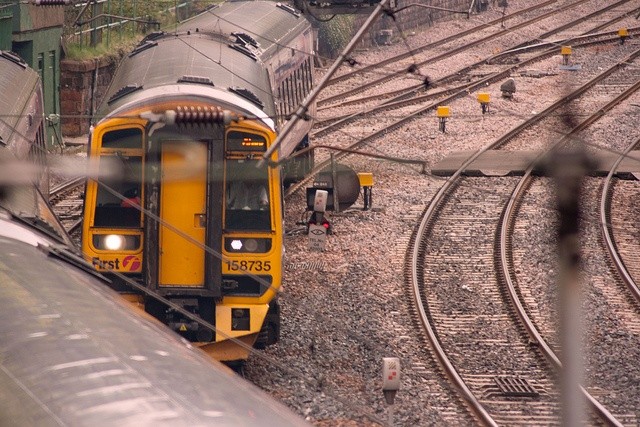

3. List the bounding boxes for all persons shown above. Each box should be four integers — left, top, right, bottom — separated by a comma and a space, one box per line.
226, 168, 269, 211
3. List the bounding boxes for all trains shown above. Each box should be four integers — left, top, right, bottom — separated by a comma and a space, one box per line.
81, 0, 316, 368
0, 49, 317, 426
